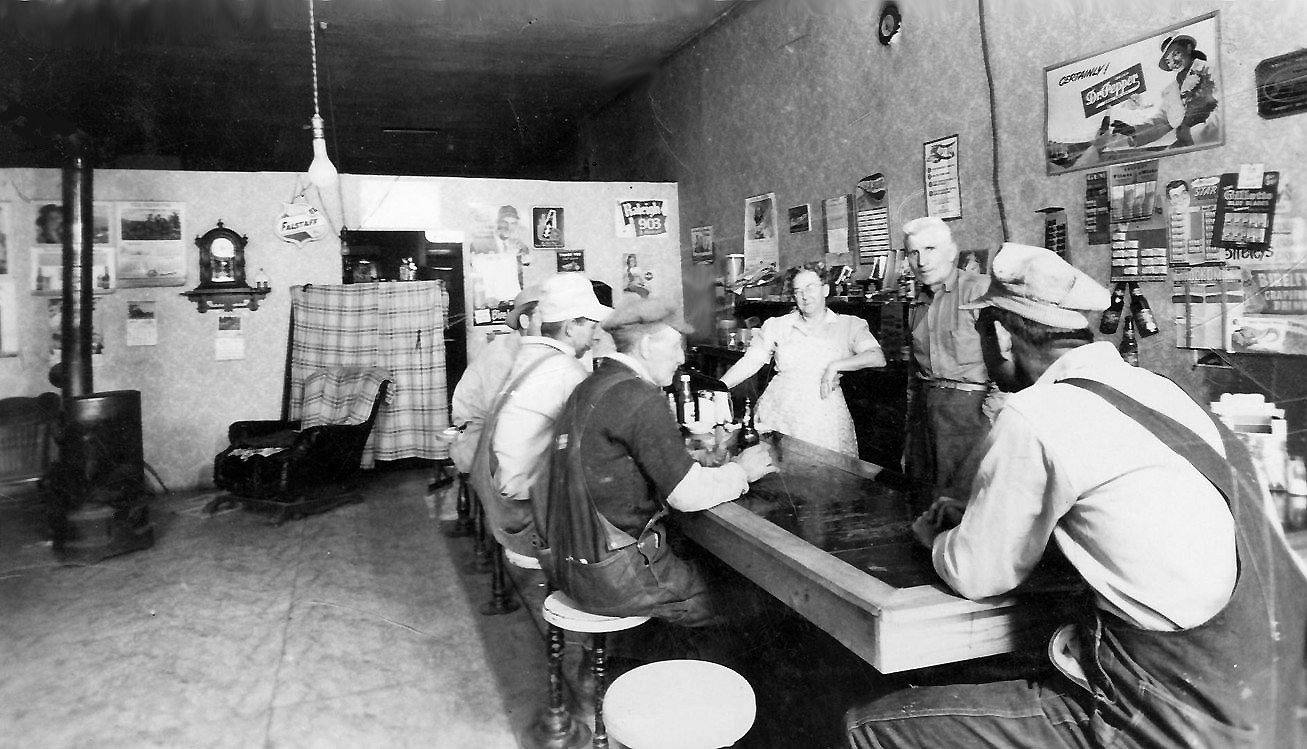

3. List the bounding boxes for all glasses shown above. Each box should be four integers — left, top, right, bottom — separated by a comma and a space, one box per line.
793, 281, 819, 298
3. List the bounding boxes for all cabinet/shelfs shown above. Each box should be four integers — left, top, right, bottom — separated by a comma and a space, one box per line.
687, 295, 922, 486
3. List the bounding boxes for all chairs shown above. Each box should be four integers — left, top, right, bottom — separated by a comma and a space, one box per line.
201, 366, 390, 529
0, 392, 64, 550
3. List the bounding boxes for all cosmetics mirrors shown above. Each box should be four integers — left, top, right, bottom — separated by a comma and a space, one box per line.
196, 220, 251, 288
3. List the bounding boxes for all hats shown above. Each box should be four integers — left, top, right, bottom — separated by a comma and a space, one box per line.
601, 297, 695, 349
957, 243, 1110, 329
505, 286, 540, 330
1159, 34, 1197, 71
540, 271, 616, 323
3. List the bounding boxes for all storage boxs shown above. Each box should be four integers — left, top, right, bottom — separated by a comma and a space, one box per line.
744, 287, 783, 297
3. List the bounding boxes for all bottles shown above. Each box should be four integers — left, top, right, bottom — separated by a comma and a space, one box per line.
255, 268, 271, 287
738, 397, 759, 456
726, 328, 761, 353
1099, 282, 1126, 334
667, 393, 678, 422
1128, 282, 1160, 338
676, 375, 696, 433
1119, 316, 1139, 367
717, 319, 737, 346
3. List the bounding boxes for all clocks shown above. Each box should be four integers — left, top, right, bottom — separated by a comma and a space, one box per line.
878, 6, 902, 45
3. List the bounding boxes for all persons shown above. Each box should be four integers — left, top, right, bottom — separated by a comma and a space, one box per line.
722, 265, 887, 460
623, 254, 653, 298
1047, 36, 1222, 175
900, 216, 1019, 487
840, 242, 1307, 749
470, 206, 531, 265
35, 204, 65, 244
631, 202, 661, 214
452, 272, 782, 749
962, 252, 981, 274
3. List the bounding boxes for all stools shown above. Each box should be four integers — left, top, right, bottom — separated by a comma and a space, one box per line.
429, 431, 756, 749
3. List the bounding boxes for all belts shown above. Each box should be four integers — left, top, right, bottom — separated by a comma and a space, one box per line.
923, 381, 988, 391
